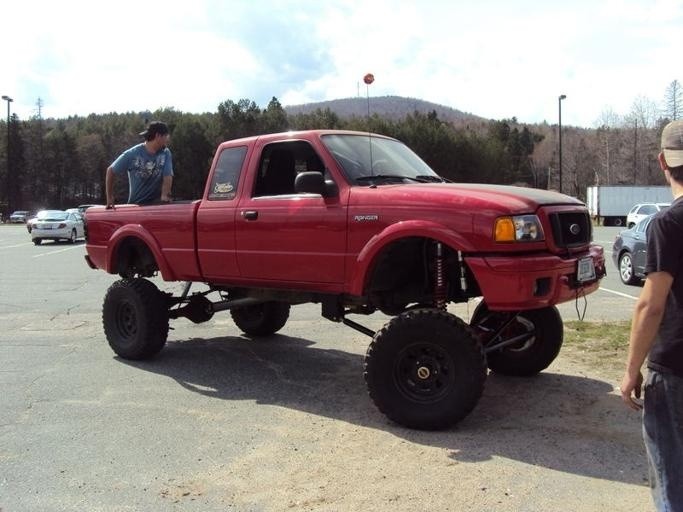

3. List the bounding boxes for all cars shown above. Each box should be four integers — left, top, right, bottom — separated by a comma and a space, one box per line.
10, 211, 31, 223
613, 213, 656, 284
26, 209, 85, 244
627, 203, 673, 230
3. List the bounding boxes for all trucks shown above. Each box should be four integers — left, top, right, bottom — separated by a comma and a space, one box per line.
587, 185, 675, 225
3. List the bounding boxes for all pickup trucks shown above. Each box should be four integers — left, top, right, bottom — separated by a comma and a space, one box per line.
83, 130, 606, 429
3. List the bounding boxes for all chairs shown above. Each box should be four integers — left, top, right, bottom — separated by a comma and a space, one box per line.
307, 149, 326, 184
255, 149, 295, 196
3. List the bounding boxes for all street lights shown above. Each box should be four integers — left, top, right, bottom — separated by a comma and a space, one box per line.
3, 96, 13, 208
559, 94, 567, 195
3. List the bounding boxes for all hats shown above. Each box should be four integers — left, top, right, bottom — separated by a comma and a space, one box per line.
661, 121, 682, 167
140, 122, 166, 136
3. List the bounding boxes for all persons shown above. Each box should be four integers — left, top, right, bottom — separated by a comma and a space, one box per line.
105, 121, 174, 211
619, 117, 683, 511
